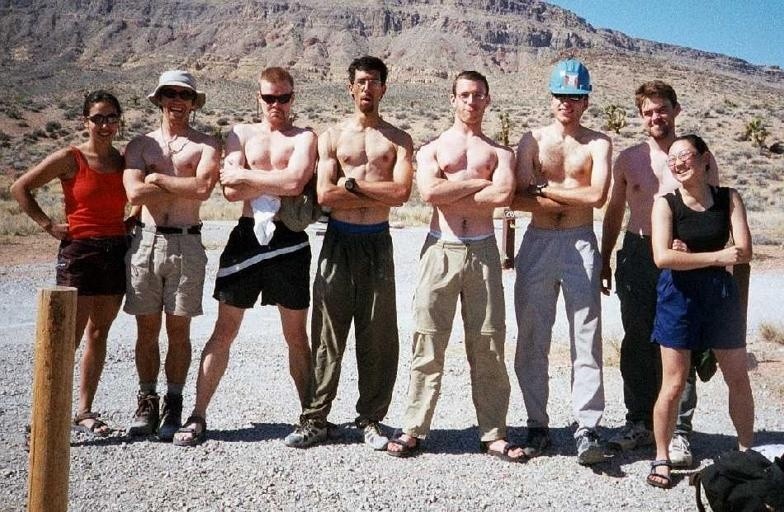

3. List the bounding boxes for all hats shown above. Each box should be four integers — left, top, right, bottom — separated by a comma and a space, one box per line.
547, 60, 592, 95
147, 71, 208, 110
280, 175, 320, 233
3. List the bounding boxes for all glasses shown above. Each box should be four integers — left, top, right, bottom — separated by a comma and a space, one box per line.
87, 113, 119, 126
552, 95, 588, 101
353, 80, 383, 90
455, 91, 488, 102
161, 87, 194, 102
665, 149, 702, 167
258, 91, 295, 104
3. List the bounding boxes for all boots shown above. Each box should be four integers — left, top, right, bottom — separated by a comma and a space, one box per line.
159, 394, 184, 441
127, 392, 159, 436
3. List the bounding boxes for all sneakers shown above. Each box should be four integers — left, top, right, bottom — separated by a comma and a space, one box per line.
669, 434, 695, 467
284, 414, 328, 449
357, 419, 389, 452
608, 422, 654, 450
545, 443, 549, 444
522, 429, 550, 457
573, 432, 605, 465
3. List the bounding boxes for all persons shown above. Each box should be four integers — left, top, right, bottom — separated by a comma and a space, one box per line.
124, 68, 219, 442
284, 57, 414, 449
600, 80, 719, 468
647, 133, 754, 491
10, 90, 144, 432
386, 71, 527, 464
173, 68, 344, 447
515, 61, 612, 468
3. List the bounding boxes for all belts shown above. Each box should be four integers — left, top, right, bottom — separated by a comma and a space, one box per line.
133, 217, 204, 238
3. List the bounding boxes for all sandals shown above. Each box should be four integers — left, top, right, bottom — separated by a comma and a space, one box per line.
172, 415, 206, 446
72, 412, 110, 437
387, 431, 421, 457
647, 459, 672, 489
302, 413, 345, 439
479, 437, 527, 462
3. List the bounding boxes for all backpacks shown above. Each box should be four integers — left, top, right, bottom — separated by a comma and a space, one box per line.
690, 449, 784, 512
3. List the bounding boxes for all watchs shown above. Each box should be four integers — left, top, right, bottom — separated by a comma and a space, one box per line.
538, 183, 547, 195
346, 177, 358, 194
